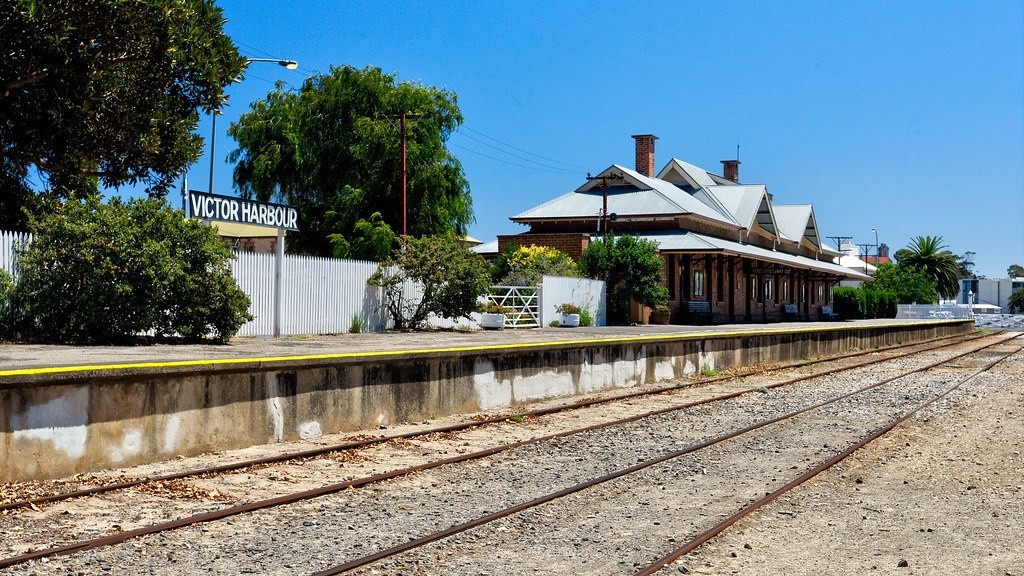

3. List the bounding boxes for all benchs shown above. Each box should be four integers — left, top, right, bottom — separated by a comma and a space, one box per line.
687, 302, 722, 324
782, 304, 805, 320
821, 305, 838, 320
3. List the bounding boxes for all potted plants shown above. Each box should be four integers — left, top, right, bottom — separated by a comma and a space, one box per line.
480, 302, 506, 330
555, 302, 581, 326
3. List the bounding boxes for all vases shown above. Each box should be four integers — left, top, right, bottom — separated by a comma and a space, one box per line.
651, 312, 671, 325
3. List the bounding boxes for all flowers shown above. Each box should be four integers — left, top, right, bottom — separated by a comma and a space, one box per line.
650, 304, 669, 312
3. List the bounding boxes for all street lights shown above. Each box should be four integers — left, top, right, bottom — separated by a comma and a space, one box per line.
872, 228, 878, 273
209, 58, 298, 195
